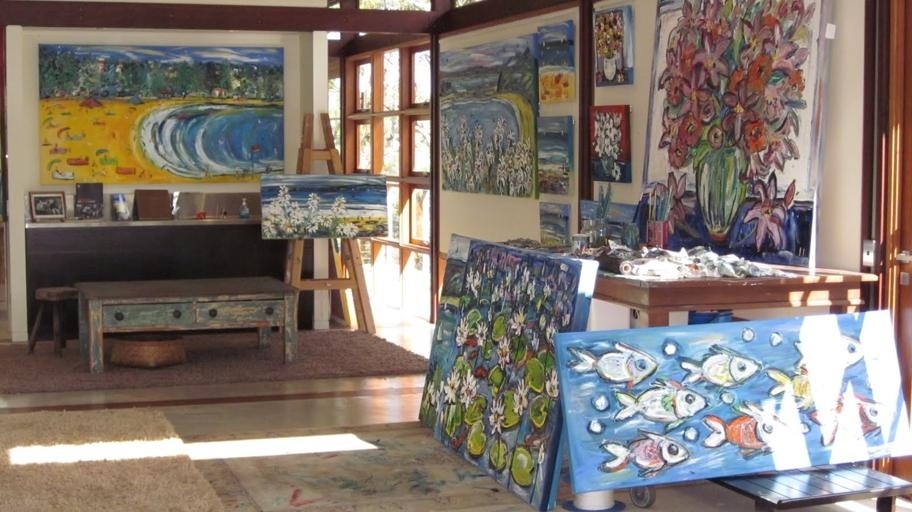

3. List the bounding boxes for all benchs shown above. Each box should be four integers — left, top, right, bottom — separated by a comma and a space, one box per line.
73, 276, 299, 371
27, 288, 77, 357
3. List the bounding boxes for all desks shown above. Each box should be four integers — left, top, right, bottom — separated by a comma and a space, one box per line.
497, 241, 865, 511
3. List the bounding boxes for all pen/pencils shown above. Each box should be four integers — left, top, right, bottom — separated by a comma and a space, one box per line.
648, 185, 672, 242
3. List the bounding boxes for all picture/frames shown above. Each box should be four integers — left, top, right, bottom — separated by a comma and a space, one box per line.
29, 191, 66, 219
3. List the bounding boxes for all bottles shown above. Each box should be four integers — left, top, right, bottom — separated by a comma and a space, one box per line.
239, 195, 250, 218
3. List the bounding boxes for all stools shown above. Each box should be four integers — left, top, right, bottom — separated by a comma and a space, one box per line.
712, 464, 912, 512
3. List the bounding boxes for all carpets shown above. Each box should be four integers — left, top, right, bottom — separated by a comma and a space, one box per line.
1, 327, 428, 394
1, 406, 224, 512
